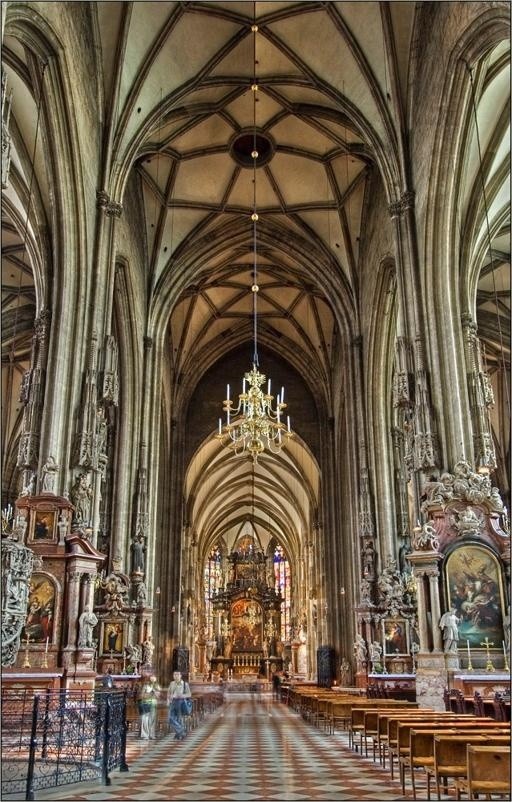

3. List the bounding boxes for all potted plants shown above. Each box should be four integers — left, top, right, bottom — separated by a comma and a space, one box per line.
126, 665, 135, 676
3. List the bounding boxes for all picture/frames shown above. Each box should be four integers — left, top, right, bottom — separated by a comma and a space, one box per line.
30, 509, 58, 541
382, 616, 411, 658
98, 619, 129, 658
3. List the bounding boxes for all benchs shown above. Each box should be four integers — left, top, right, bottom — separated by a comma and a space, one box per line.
121, 688, 223, 732
271, 679, 511, 800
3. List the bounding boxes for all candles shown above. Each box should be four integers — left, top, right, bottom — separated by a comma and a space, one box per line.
2, 504, 20, 532
233, 655, 260, 666
228, 668, 229, 674
46, 636, 49, 653
231, 669, 233, 675
502, 640, 507, 657
466, 639, 471, 666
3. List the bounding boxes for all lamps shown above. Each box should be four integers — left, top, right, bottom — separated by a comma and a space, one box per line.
218, 120, 295, 455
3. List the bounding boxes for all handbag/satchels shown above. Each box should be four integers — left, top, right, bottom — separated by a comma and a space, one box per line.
137, 704, 151, 715
181, 700, 193, 717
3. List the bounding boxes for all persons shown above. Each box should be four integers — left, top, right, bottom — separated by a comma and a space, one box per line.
78, 605, 99, 647
438, 608, 464, 652
27, 597, 42, 625
36, 516, 49, 539
140, 673, 161, 740
167, 670, 191, 739
